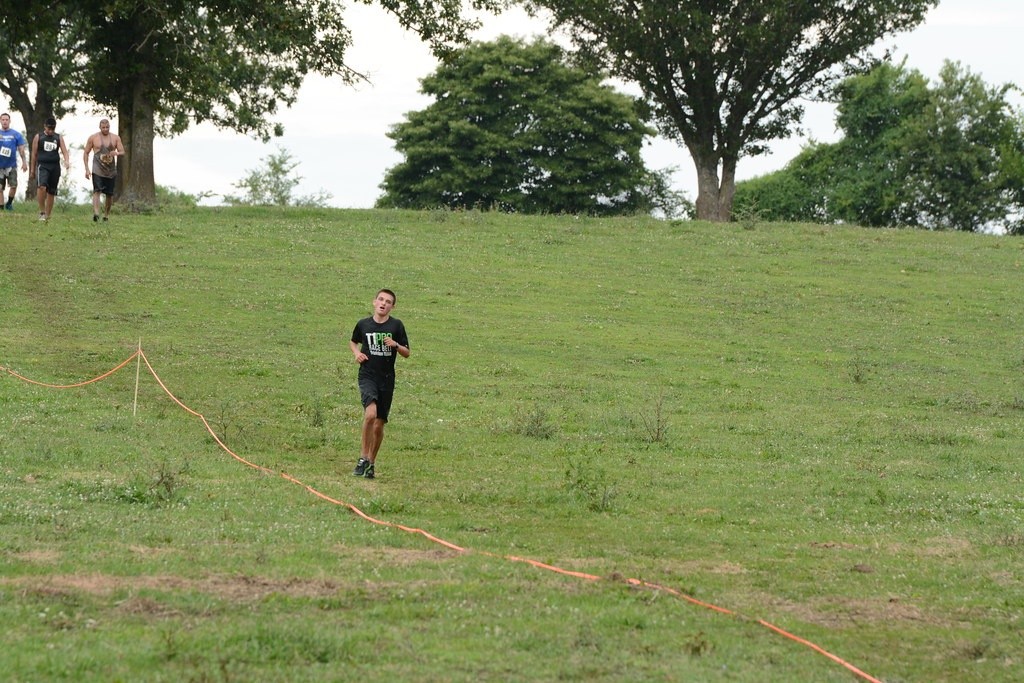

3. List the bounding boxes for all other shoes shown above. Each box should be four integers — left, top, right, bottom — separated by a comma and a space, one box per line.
38, 212, 45, 220
6, 204, 13, 210
94, 215, 99, 221
104, 217, 108, 221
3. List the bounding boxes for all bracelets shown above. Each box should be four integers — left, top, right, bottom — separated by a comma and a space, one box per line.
395, 342, 399, 349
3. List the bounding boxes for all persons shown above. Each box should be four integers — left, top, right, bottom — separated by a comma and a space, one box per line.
84, 119, 125, 222
29, 115, 69, 222
0, 113, 28, 210
349, 289, 411, 479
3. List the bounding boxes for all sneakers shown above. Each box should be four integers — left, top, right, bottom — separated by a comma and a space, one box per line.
352, 458, 371, 477
364, 463, 374, 479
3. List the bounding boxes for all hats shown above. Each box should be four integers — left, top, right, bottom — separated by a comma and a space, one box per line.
46, 118, 56, 128
